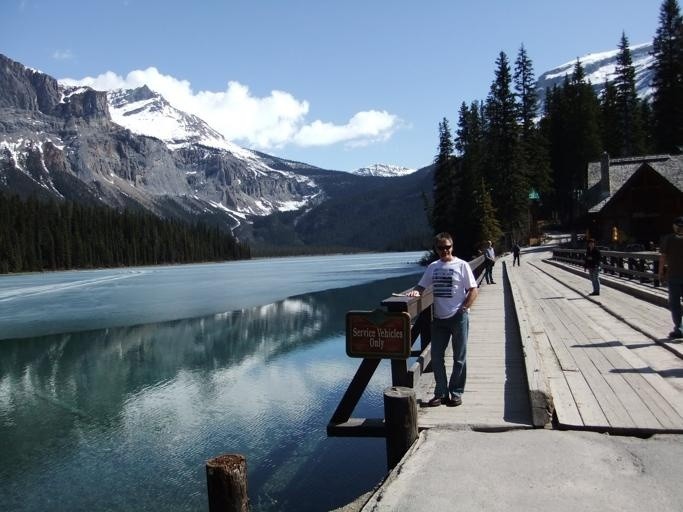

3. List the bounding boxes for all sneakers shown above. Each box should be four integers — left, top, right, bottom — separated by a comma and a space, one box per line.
589, 290, 600, 296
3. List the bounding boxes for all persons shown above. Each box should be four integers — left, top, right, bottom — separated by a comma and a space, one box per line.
583, 239, 601, 295
657, 216, 683, 339
405, 230, 477, 407
483, 240, 497, 285
510, 243, 520, 266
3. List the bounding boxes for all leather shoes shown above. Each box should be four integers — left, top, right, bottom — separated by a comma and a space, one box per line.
429, 395, 462, 407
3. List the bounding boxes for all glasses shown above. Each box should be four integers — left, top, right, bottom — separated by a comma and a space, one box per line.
435, 245, 453, 249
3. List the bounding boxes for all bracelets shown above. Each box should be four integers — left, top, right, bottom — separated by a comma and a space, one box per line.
460, 306, 467, 312
